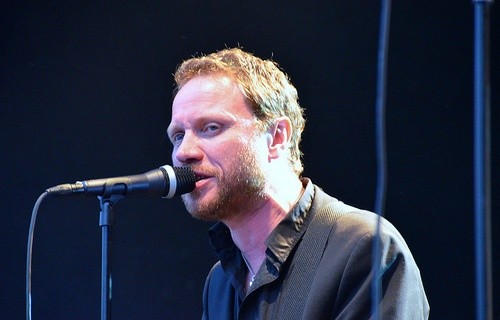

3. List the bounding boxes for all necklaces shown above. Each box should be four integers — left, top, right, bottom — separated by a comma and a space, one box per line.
239, 251, 261, 285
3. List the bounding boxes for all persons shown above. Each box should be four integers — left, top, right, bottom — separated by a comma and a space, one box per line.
165, 48, 432, 320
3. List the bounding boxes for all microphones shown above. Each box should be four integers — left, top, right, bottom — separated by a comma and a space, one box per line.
45, 164, 197, 201
